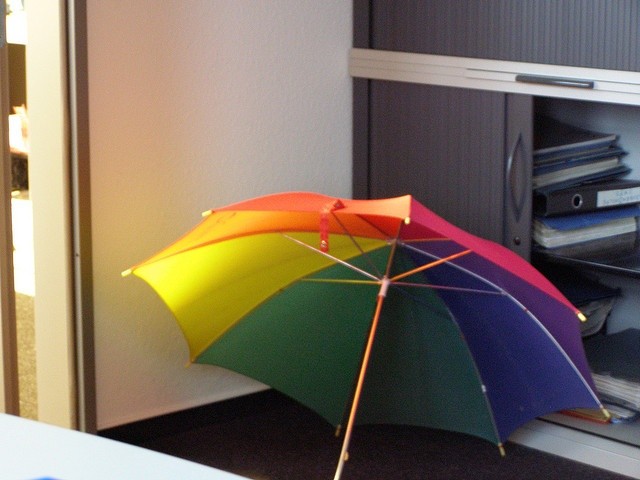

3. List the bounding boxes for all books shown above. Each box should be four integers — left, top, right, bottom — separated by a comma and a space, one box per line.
529, 264, 640, 425
532, 111, 640, 250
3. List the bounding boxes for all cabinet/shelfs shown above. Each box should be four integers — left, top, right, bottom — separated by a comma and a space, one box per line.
350, 1, 637, 94
355, 77, 640, 480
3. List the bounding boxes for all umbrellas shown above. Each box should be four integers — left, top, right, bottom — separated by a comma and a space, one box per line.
121, 190, 614, 480
